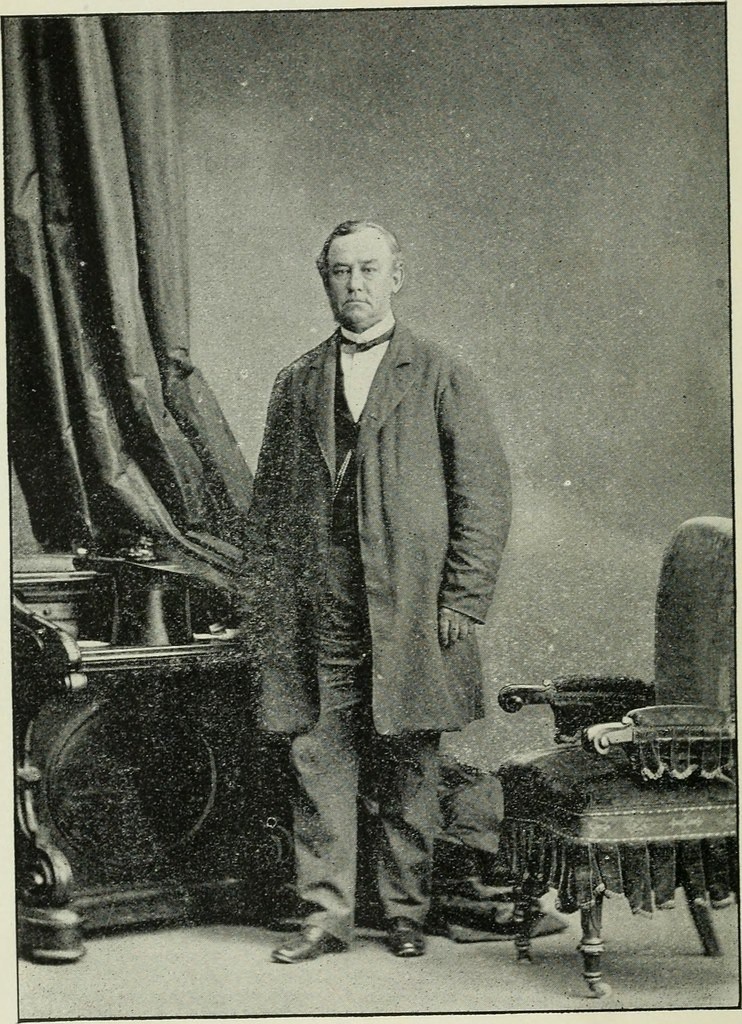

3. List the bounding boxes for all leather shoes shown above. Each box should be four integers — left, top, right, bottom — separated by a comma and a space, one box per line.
271, 927, 345, 965
389, 914, 424, 956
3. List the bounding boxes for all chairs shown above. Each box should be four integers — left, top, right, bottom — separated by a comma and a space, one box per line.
493, 515, 739, 1000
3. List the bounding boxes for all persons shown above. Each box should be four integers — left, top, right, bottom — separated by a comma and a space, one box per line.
233, 221, 515, 962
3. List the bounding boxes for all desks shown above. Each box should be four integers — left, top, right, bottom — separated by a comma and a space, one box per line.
34, 639, 304, 932
